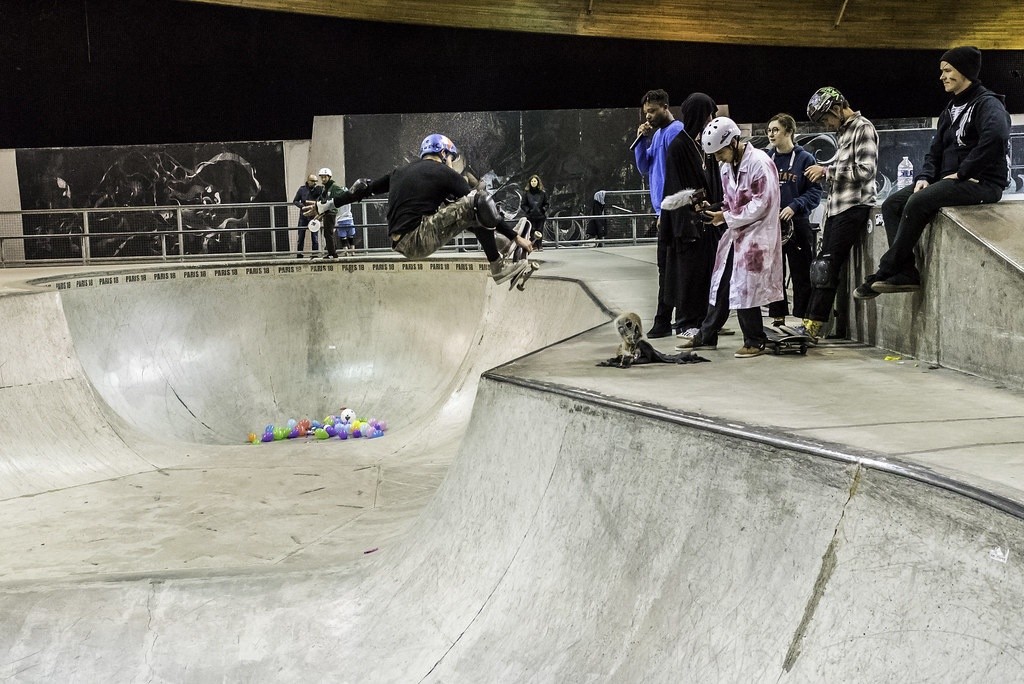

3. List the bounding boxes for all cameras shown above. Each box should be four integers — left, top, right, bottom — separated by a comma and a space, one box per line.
688, 188, 724, 226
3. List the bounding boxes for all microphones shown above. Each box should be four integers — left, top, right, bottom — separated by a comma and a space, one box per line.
627, 121, 651, 152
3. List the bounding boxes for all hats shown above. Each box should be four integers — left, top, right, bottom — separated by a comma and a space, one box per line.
940, 45, 983, 81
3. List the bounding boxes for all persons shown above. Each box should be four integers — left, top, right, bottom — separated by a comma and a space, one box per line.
630, 88, 686, 338
660, 94, 736, 340
673, 117, 784, 357
520, 174, 550, 251
769, 87, 881, 348
591, 190, 608, 247
315, 167, 345, 259
292, 174, 324, 259
334, 185, 357, 257
851, 45, 1012, 301
763, 113, 824, 336
302, 132, 535, 285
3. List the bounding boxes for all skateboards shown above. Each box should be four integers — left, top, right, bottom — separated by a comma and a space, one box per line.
761, 324, 811, 355
509, 220, 542, 291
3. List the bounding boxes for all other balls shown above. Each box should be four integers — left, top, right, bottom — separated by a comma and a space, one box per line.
247, 408, 387, 446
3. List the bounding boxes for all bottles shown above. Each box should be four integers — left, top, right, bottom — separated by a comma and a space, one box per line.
897, 156, 914, 189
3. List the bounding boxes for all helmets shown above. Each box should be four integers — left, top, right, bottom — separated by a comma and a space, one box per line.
419, 134, 460, 162
806, 88, 846, 123
319, 168, 332, 176
702, 117, 741, 154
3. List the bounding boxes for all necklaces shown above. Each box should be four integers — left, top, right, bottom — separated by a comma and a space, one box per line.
682, 128, 707, 170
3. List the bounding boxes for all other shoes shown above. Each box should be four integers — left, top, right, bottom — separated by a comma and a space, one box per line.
295, 239, 356, 259
675, 303, 835, 358
533, 243, 538, 252
538, 240, 543, 251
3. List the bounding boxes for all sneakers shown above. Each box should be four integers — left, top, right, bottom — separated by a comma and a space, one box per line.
853, 273, 886, 299
871, 268, 921, 296
490, 256, 528, 284
494, 217, 528, 255
676, 328, 701, 338
647, 315, 673, 338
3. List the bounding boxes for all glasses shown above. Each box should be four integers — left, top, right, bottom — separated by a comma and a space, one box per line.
309, 180, 319, 182
765, 127, 789, 136
